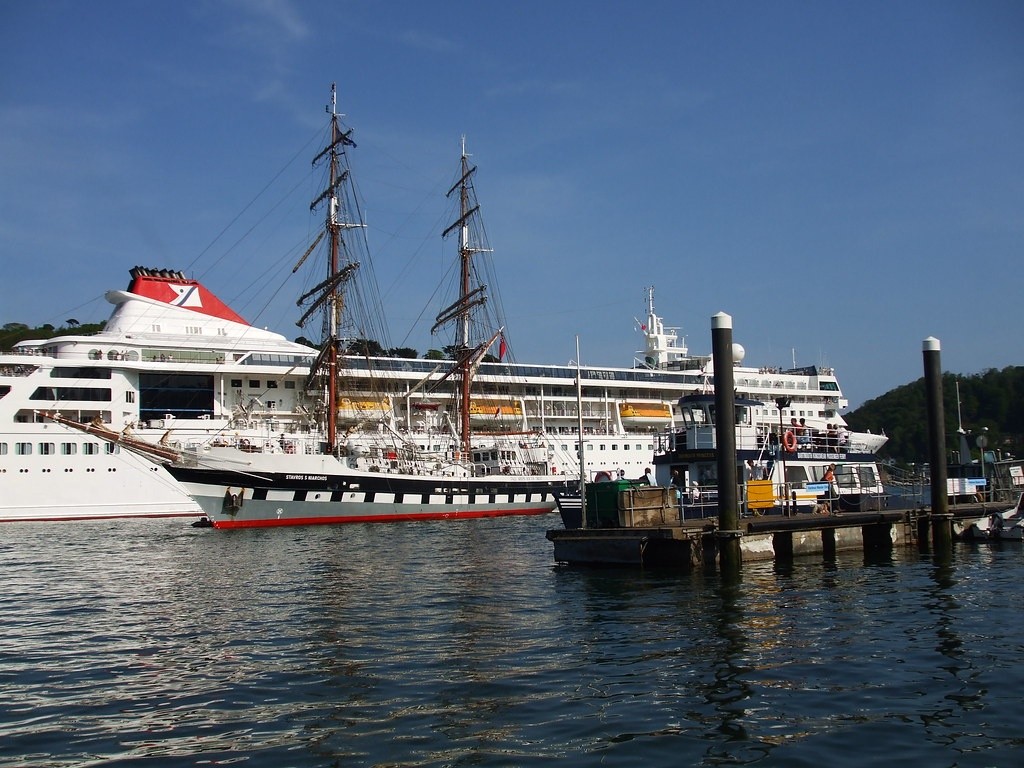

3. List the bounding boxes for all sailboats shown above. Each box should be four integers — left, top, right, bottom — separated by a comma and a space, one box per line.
32, 80, 616, 530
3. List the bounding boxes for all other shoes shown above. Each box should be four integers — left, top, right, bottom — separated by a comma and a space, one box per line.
833, 510, 839, 513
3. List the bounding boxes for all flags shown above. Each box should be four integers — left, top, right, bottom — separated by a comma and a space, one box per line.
642, 324, 646, 330
499, 337, 505, 361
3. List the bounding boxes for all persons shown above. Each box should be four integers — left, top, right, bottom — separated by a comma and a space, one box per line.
121, 350, 124, 360
820, 464, 844, 512
639, 468, 658, 487
747, 457, 769, 481
790, 418, 810, 438
41, 346, 48, 356
95, 350, 104, 361
160, 353, 174, 363
219, 356, 223, 362
826, 423, 845, 443
691, 481, 700, 502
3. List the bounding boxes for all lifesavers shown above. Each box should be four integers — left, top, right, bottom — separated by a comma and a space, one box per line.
968, 493, 984, 503
783, 431, 798, 452
595, 471, 612, 483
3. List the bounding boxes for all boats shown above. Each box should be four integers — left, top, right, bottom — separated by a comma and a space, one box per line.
543, 341, 1024, 571
0, 262, 890, 521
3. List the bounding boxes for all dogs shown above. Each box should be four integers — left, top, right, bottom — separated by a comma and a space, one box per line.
810, 501, 831, 515
